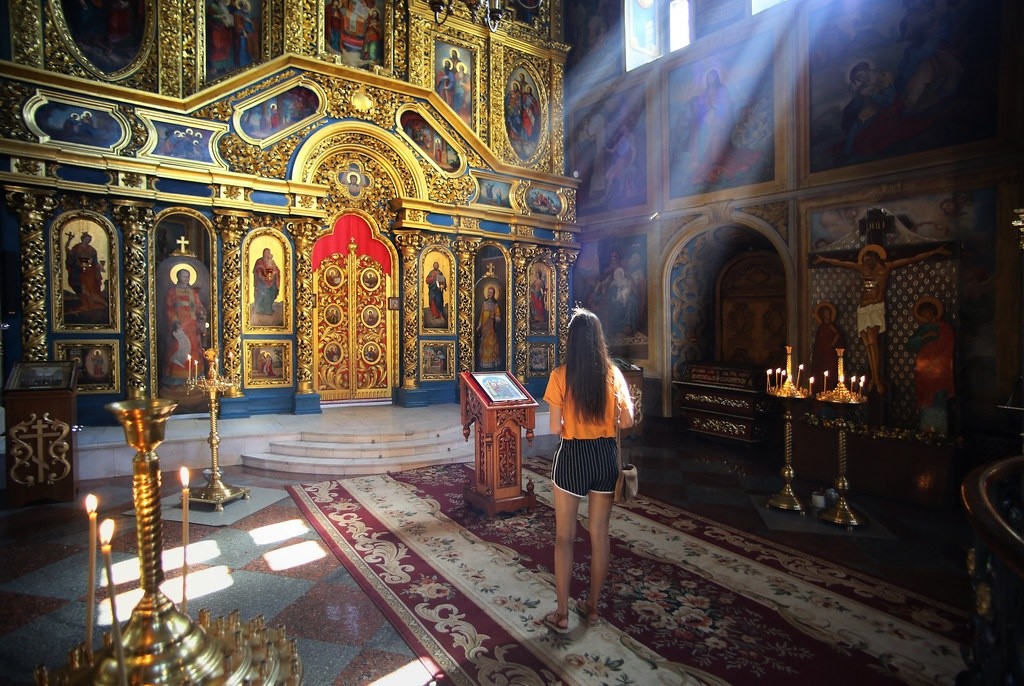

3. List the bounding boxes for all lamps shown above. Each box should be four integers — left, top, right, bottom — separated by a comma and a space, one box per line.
426, 0, 543, 33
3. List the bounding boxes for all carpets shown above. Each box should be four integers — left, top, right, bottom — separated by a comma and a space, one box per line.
749, 494, 899, 541
285, 455, 976, 686
121, 481, 290, 526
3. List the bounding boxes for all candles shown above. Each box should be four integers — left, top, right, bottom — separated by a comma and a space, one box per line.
766, 368, 786, 391
851, 376, 856, 393
98, 518, 128, 686
810, 376, 814, 395
230, 352, 234, 384
797, 363, 804, 389
194, 359, 198, 382
858, 374, 865, 396
187, 353, 191, 380
84, 492, 98, 663
824, 370, 829, 392
180, 466, 190, 614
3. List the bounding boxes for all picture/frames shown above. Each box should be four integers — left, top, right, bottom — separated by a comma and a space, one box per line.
312, 293, 317, 308
388, 296, 399, 310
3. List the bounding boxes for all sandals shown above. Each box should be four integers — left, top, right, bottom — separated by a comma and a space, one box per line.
577, 599, 600, 624
543, 611, 570, 633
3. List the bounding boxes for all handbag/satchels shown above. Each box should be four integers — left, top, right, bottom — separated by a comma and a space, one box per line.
613, 464, 638, 503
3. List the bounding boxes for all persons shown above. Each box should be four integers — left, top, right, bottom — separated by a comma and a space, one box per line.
542, 310, 634, 633
